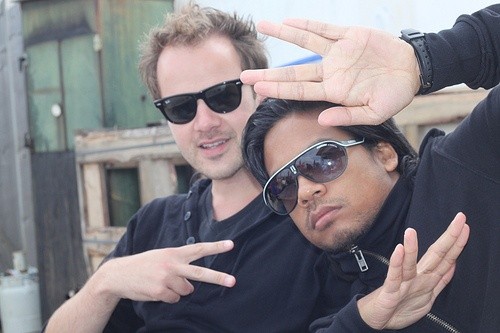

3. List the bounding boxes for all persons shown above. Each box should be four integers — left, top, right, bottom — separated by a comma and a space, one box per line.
43, 1, 323, 332
238, 3, 500, 333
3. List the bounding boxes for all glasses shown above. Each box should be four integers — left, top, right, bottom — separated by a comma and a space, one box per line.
154, 78, 243, 124
261, 137, 365, 216
